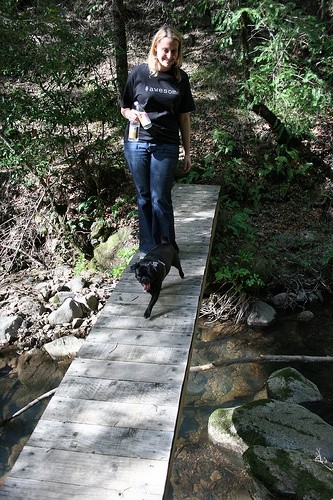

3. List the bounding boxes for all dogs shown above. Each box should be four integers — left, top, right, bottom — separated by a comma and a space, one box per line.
130, 233, 184, 319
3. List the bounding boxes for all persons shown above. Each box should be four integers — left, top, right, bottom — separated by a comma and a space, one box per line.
120, 24, 195, 260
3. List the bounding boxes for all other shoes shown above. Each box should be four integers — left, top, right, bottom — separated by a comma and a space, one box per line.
139, 252, 146, 261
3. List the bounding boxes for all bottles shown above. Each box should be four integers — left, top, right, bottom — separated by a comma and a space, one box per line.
128, 120, 140, 142
134, 101, 152, 130
